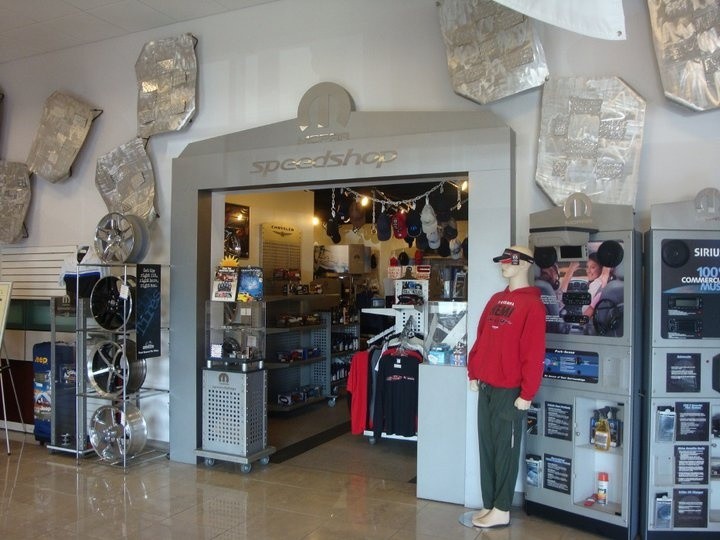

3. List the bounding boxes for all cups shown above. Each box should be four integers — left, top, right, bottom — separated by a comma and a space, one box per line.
386, 296, 394, 308
303, 285, 308, 294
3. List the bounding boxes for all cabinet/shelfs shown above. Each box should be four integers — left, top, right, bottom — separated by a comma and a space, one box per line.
264, 292, 361, 413
77, 259, 170, 473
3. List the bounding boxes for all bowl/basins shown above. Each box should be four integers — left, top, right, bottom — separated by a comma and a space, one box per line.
370, 298, 384, 308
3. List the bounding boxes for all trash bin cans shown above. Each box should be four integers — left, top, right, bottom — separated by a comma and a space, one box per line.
33, 342, 76, 445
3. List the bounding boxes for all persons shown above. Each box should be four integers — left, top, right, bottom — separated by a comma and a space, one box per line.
466, 246, 545, 526
582, 240, 625, 335
531, 246, 580, 334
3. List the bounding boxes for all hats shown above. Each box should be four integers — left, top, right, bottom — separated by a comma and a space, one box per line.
399, 253, 409, 265
377, 214, 392, 242
415, 250, 423, 265
449, 238, 462, 260
390, 257, 398, 267
443, 225, 458, 240
350, 203, 365, 233
406, 209, 421, 237
420, 205, 440, 249
493, 249, 535, 266
462, 238, 468, 259
327, 218, 341, 243
336, 213, 350, 224
438, 238, 451, 257
402, 235, 415, 248
392, 211, 408, 239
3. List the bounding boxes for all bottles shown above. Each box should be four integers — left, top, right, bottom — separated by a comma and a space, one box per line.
331, 362, 350, 381
332, 333, 352, 353
598, 473, 608, 505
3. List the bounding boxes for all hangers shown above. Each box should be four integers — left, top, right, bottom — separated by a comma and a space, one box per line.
381, 320, 424, 367
